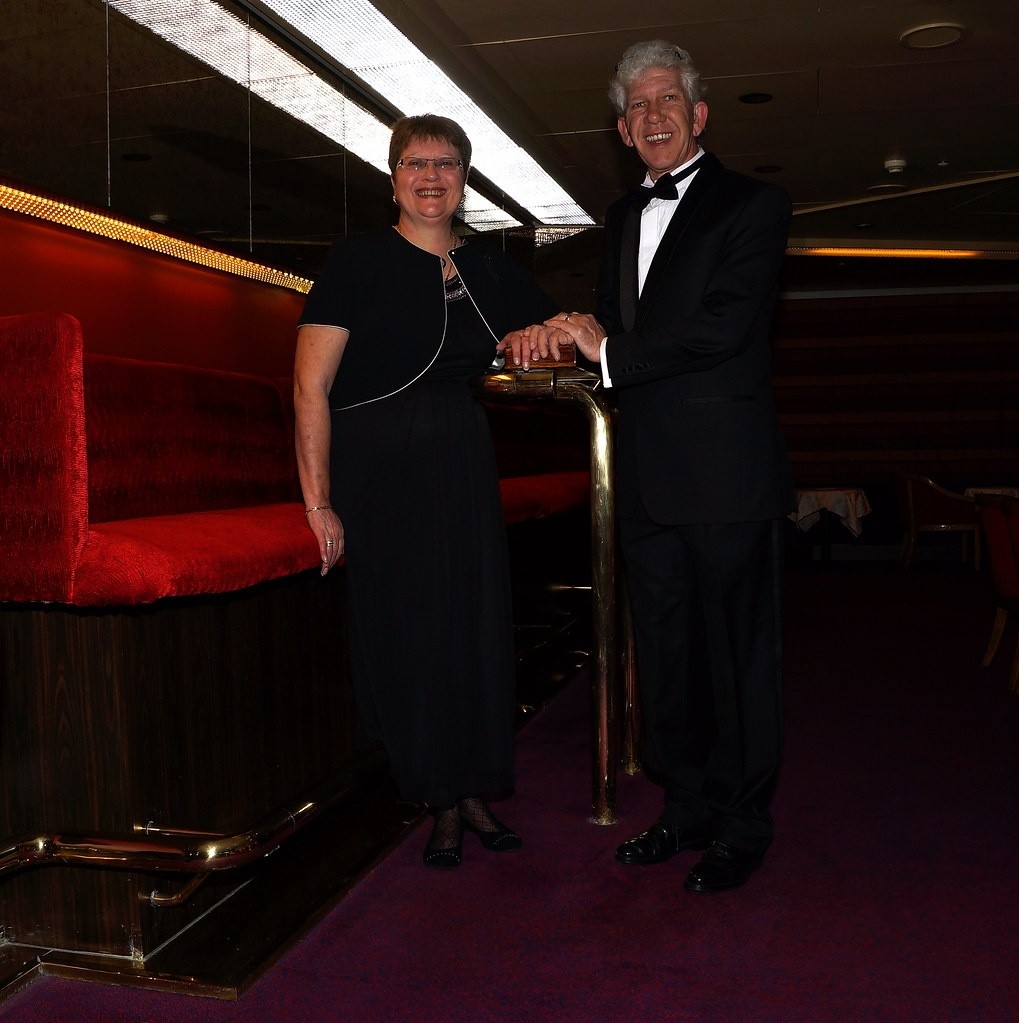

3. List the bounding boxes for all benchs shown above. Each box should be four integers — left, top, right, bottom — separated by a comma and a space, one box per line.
278, 375, 621, 716
0, 309, 378, 1000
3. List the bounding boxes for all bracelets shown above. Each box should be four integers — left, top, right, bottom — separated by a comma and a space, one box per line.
305, 505, 333, 513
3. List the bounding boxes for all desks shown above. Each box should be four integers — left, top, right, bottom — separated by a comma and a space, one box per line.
786, 486, 872, 580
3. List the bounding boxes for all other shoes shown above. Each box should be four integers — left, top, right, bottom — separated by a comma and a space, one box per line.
462, 804, 523, 853
424, 814, 460, 873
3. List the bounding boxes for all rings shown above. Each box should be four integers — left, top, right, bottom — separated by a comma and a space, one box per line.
564, 314, 572, 321
327, 541, 333, 547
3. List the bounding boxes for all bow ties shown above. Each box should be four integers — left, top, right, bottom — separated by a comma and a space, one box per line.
626, 154, 707, 212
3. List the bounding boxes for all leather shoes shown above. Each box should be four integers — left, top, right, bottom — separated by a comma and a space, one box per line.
682, 827, 773, 893
616, 818, 720, 865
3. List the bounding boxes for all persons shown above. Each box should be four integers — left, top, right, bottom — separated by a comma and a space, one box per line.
293, 116, 541, 867
523, 40, 793, 895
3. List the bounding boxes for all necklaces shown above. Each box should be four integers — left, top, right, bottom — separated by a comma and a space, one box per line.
444, 231, 457, 281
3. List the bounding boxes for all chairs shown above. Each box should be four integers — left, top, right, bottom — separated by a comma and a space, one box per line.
965, 487, 1019, 699
904, 475, 982, 569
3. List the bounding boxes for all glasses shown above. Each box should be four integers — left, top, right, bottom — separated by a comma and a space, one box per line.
394, 158, 467, 173
